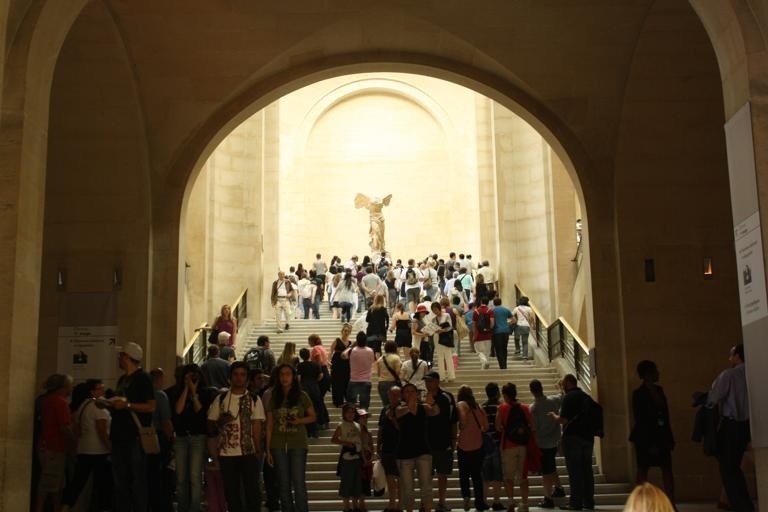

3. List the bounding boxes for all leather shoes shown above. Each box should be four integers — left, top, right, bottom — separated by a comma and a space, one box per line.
560, 503, 582, 511
582, 503, 595, 511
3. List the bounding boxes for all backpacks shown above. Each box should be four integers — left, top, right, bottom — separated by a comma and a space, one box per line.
405, 267, 419, 285
475, 307, 492, 335
243, 348, 264, 372
505, 403, 532, 445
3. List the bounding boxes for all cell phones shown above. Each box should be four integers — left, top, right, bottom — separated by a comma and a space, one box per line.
224, 412, 232, 417
400, 401, 408, 406
285, 416, 293, 426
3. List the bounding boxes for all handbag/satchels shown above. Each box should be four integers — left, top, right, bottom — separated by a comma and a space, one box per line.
437, 322, 457, 348
422, 278, 433, 290
480, 430, 497, 454
208, 329, 219, 345
139, 422, 162, 455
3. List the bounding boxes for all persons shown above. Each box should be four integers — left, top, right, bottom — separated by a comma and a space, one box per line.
30, 252, 757, 510
368, 192, 387, 250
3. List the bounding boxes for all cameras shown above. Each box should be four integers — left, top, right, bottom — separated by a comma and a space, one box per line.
191, 374, 199, 384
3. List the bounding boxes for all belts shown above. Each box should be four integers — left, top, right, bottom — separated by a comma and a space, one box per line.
277, 295, 290, 299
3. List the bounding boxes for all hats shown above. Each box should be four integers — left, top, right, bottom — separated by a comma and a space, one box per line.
414, 305, 429, 315
356, 407, 373, 418
218, 330, 232, 344
113, 341, 144, 363
421, 370, 440, 382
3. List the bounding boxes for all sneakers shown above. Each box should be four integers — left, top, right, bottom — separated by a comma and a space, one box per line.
464, 496, 471, 512
536, 496, 555, 509
441, 376, 456, 383
491, 502, 507, 511
508, 499, 518, 512
514, 350, 529, 361
475, 499, 490, 512
516, 501, 529, 512
435, 501, 452, 512
551, 486, 566, 498
342, 505, 368, 512
483, 362, 490, 369
383, 502, 433, 512
